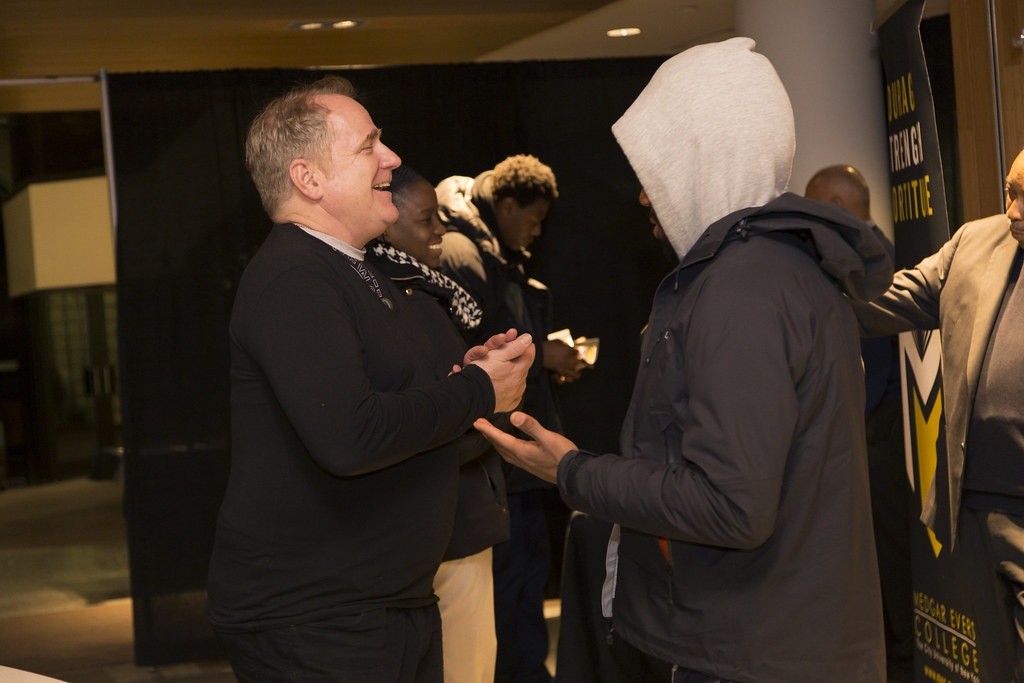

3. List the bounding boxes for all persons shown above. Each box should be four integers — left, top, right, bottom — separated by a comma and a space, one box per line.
804, 165, 914, 683
434, 154, 581, 683
851, 147, 1024, 683
473, 37, 895, 683
207, 73, 536, 682
358, 167, 511, 683
